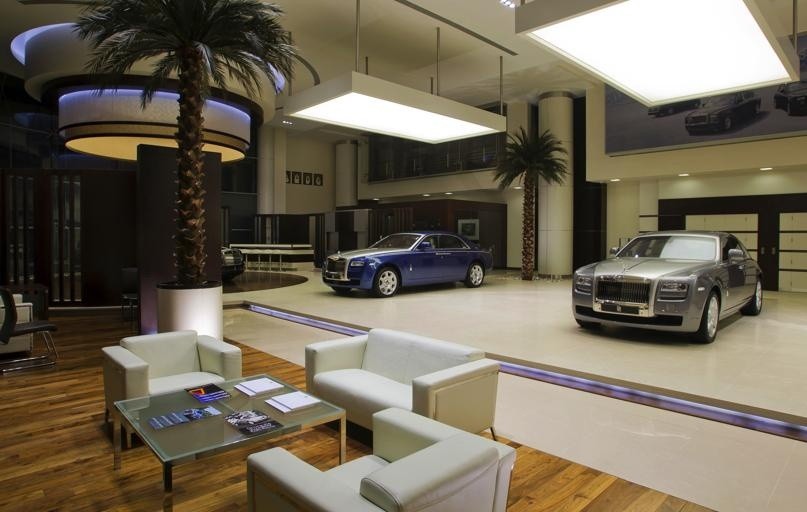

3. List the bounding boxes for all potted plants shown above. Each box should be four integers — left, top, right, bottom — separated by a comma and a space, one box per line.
71, 0, 296, 343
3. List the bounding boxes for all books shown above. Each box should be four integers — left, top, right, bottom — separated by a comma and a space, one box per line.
233, 383, 279, 398
239, 377, 284, 393
271, 391, 320, 410
183, 382, 232, 405
263, 397, 314, 415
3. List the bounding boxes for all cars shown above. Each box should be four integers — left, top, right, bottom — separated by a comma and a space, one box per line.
645, 98, 706, 118
233, 412, 268, 427
772, 79, 807, 117
319, 228, 486, 300
681, 89, 766, 137
220, 242, 247, 283
570, 229, 771, 347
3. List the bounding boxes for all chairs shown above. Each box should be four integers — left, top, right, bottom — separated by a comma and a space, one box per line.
100, 328, 244, 453
0, 282, 61, 376
